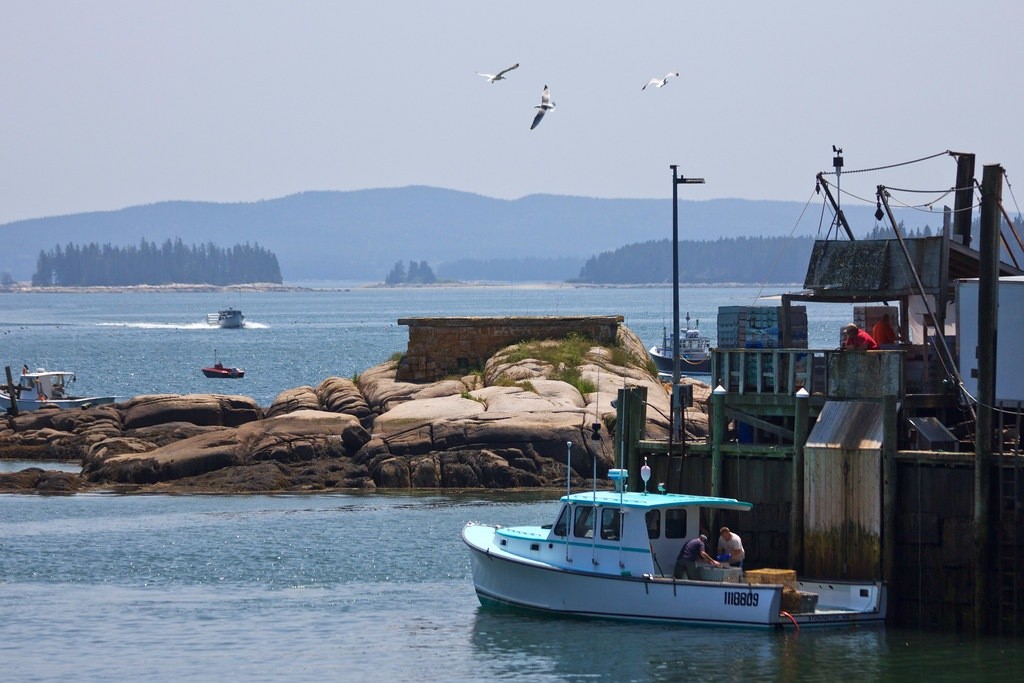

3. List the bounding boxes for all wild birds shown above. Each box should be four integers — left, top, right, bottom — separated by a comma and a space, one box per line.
641, 69, 682, 92
474, 63, 557, 131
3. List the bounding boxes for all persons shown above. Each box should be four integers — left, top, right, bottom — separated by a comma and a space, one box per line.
840, 323, 879, 351
673, 535, 722, 580
717, 527, 746, 566
871, 313, 897, 345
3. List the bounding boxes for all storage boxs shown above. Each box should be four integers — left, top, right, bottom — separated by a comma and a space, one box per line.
716, 305, 807, 386
698, 567, 741, 584
744, 568, 796, 594
853, 305, 897, 339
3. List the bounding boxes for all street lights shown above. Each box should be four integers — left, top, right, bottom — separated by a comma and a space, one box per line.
669, 176, 708, 439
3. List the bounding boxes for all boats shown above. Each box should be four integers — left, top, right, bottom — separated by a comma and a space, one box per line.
201, 349, 244, 378
460, 312, 889, 626
0, 365, 122, 413
206, 305, 246, 328
649, 312, 715, 376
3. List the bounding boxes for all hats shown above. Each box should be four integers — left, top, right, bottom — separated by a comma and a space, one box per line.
843, 324, 857, 333
